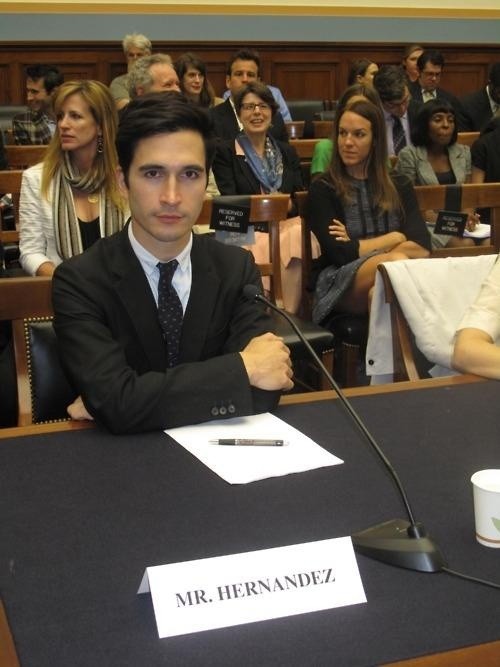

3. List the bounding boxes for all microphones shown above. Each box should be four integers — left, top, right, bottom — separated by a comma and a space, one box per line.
242, 283, 449, 573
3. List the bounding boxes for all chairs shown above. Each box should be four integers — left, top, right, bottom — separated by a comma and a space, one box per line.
0, 92, 499, 431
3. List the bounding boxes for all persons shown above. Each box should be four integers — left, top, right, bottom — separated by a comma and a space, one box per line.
49, 90, 295, 438
449, 249, 500, 383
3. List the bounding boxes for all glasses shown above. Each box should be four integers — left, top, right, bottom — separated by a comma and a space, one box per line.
240, 103, 269, 110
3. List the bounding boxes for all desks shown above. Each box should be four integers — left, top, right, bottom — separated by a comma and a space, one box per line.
0, 366, 499, 665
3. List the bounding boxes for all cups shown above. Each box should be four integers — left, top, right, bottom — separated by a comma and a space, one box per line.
472, 467, 500, 547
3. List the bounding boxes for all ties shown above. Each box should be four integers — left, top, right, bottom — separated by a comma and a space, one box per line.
156, 259, 184, 369
390, 114, 406, 156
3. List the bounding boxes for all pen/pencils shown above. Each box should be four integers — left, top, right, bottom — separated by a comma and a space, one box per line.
207, 439, 283, 446
